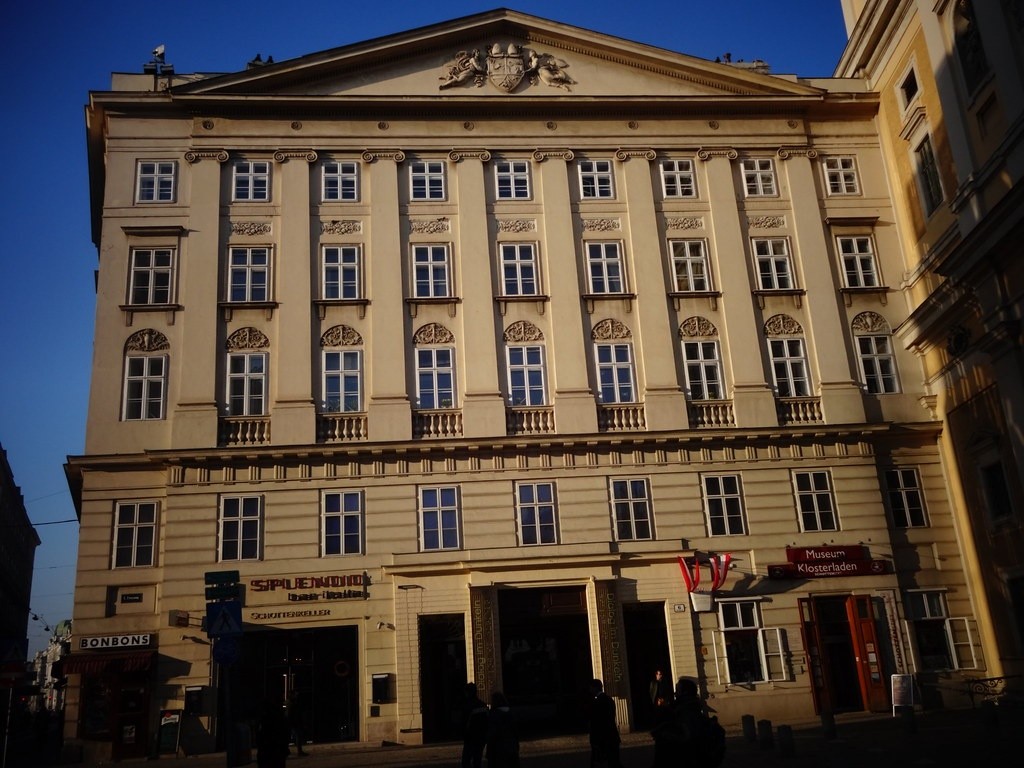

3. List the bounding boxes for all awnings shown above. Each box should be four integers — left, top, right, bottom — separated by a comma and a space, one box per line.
53, 651, 158, 673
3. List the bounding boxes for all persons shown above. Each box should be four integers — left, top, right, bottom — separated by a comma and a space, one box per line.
258, 690, 310, 768
649, 668, 666, 711
651, 680, 704, 768
224, 710, 254, 768
461, 691, 519, 768
587, 679, 622, 768
